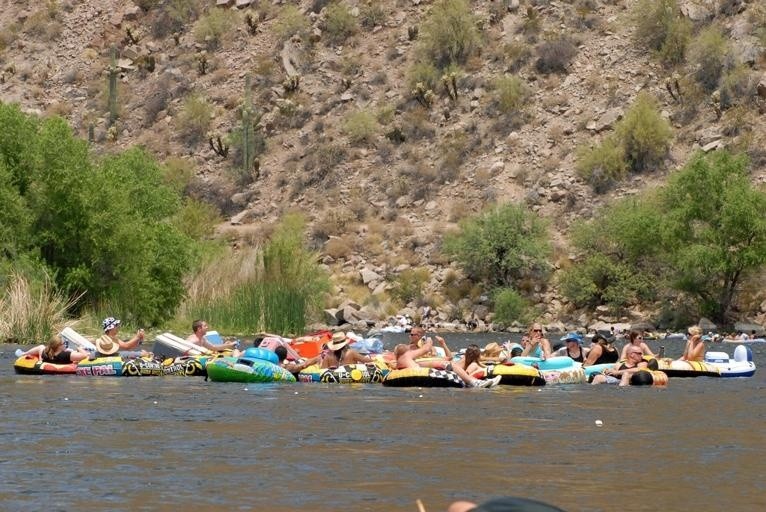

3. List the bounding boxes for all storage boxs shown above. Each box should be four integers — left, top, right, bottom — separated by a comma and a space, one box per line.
205, 330, 224, 345
289, 334, 329, 359
258, 337, 296, 361
705, 351, 729, 363
152, 333, 209, 361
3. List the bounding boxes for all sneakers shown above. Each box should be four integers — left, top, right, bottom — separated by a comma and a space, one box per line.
471, 375, 502, 388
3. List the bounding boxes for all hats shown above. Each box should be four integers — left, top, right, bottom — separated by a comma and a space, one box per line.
102, 317, 120, 332
327, 331, 350, 350
560, 333, 584, 344
597, 330, 616, 343
688, 326, 703, 335
96, 335, 119, 355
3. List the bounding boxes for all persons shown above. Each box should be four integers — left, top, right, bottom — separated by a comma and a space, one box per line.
90, 334, 148, 358
16, 335, 88, 364
188, 319, 235, 351
253, 332, 322, 372
102, 318, 144, 349
392, 323, 551, 388
553, 328, 659, 385
322, 331, 372, 369
678, 325, 705, 360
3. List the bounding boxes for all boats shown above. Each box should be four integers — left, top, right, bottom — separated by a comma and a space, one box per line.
702, 333, 724, 343
558, 326, 617, 345
13, 354, 78, 374
641, 345, 757, 378
657, 333, 686, 340
623, 330, 658, 340
75, 329, 396, 378
205, 347, 297, 383
723, 335, 766, 345
298, 356, 389, 383
433, 340, 669, 387
381, 367, 467, 388
